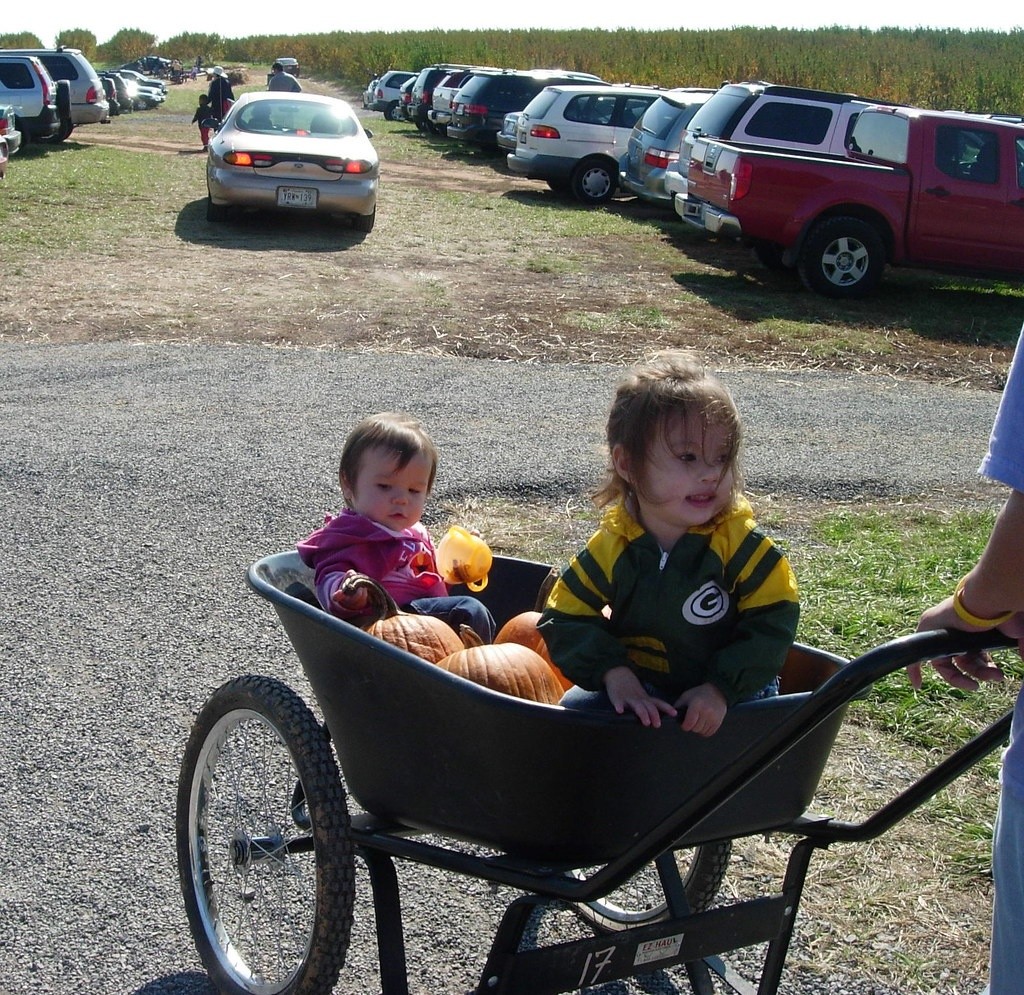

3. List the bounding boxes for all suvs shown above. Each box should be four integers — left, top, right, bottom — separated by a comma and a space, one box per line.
618, 88, 720, 205
664, 79, 1024, 198
0, 45, 111, 144
0, 56, 72, 147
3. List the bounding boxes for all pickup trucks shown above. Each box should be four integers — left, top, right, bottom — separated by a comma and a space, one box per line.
674, 105, 1024, 299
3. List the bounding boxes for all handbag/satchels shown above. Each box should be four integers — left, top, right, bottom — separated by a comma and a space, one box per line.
224, 101, 231, 113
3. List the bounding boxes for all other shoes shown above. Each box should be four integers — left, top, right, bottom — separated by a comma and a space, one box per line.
202, 146, 209, 152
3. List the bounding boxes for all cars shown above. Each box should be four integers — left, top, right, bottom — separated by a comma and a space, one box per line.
496, 97, 649, 153
507, 86, 668, 205
120, 56, 172, 75
446, 69, 612, 150
96, 70, 168, 116
0, 103, 22, 155
271, 58, 300, 78
360, 63, 504, 124
200, 91, 379, 232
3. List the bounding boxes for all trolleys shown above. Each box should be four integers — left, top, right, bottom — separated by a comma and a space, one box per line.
176, 545, 1020, 995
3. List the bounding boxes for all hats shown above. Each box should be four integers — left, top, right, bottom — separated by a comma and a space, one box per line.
214, 66, 228, 79
273, 63, 284, 70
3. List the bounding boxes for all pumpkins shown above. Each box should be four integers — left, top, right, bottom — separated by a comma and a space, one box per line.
345, 568, 581, 707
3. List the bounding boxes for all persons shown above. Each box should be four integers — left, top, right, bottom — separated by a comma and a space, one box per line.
297, 412, 497, 646
191, 94, 217, 152
190, 66, 197, 81
206, 66, 235, 133
195, 55, 202, 73
909, 313, 1024, 993
535, 349, 799, 739
172, 60, 182, 84
268, 62, 302, 93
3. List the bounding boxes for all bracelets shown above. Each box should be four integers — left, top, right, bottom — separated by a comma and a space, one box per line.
953, 576, 1014, 626
